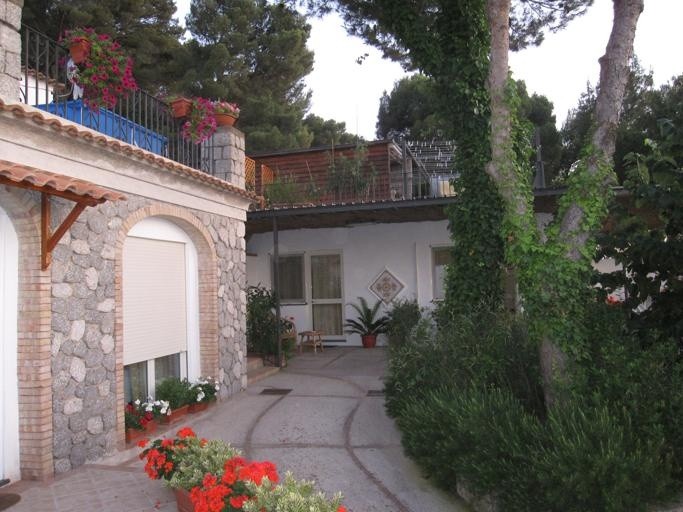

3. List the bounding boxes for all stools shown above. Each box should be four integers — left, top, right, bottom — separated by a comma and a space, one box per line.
298, 331, 324, 355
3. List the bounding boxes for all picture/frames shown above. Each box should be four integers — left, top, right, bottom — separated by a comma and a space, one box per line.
368, 265, 406, 308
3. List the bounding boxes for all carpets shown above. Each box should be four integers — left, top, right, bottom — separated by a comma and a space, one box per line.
0, 494, 21, 512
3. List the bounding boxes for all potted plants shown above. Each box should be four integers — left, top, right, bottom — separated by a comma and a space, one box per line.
266, 171, 317, 210
342, 297, 392, 347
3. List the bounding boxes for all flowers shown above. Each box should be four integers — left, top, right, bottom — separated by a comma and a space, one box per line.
211, 97, 240, 118
125, 375, 348, 512
57, 28, 138, 115
158, 94, 217, 144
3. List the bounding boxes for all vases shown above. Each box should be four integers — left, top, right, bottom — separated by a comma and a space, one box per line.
173, 98, 193, 118
213, 113, 238, 127
69, 37, 90, 63
